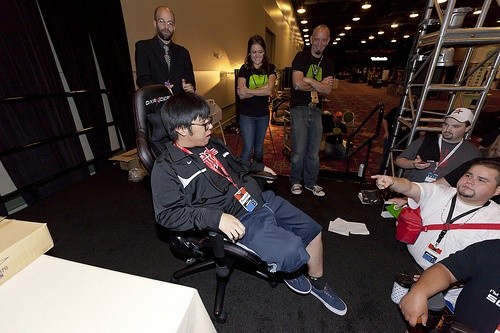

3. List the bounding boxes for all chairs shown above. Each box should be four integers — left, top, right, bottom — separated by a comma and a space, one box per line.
131, 84, 278, 324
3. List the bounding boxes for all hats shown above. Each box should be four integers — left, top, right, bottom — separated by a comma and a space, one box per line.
443, 108, 474, 129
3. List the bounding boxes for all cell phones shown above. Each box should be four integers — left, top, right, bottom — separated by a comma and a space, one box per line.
417, 160, 435, 164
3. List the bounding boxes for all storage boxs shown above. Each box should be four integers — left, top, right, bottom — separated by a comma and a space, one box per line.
108, 148, 143, 170
0, 216, 56, 286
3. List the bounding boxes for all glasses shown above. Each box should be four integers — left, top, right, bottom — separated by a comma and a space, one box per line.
190, 115, 214, 130
154, 18, 176, 26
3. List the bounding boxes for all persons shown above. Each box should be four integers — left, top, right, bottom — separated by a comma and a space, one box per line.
399, 239, 499, 333
369, 158, 500, 271
151, 92, 349, 315
235, 34, 277, 166
394, 107, 478, 183
135, 6, 197, 93
289, 24, 334, 195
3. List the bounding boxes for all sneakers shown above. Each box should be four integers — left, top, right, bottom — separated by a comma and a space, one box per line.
281, 269, 312, 295
290, 183, 302, 194
310, 281, 348, 316
305, 183, 325, 197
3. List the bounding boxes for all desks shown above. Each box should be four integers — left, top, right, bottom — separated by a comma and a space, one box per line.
1, 253, 221, 332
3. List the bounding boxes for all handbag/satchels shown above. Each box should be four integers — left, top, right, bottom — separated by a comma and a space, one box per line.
395, 206, 423, 245
